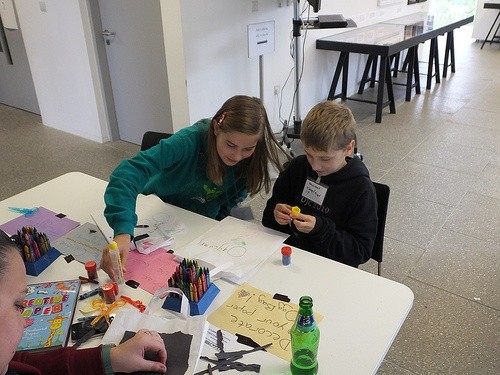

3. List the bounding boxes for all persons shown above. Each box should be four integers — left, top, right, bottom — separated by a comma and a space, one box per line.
262, 101, 378, 269
0, 230, 167, 375
99, 95, 293, 285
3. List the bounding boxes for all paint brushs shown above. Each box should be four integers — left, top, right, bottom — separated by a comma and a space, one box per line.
79, 286, 103, 300
168, 257, 210, 302
79, 275, 99, 284
16, 225, 51, 261
77, 315, 98, 322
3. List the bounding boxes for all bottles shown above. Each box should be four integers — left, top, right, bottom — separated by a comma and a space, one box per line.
290, 296, 320, 375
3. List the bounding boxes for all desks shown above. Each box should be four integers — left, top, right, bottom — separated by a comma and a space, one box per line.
315, 10, 473, 123
0, 155, 413, 375
480, 4, 500, 49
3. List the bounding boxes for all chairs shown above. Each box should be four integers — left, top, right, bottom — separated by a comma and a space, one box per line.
352, 181, 392, 279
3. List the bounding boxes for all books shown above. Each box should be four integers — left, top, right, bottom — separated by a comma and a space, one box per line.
16, 278, 81, 353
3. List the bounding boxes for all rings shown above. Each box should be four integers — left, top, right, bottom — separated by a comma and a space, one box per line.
144, 332, 151, 335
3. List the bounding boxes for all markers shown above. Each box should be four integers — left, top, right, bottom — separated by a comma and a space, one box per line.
134, 224, 150, 229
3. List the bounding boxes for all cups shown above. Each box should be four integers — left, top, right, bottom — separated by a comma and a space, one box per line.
426, 15, 435, 30
